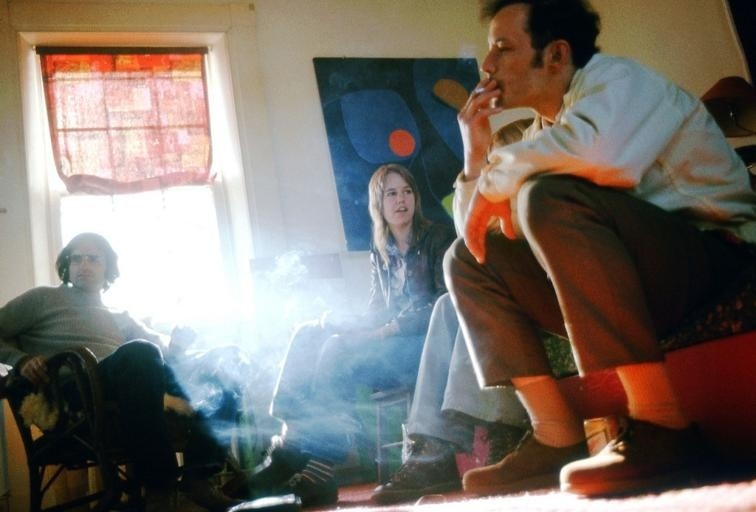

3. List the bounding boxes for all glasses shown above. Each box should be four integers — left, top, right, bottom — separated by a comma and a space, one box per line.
64, 252, 105, 266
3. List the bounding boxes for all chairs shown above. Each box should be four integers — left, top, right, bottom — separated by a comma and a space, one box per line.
368, 378, 420, 483
544, 72, 756, 481
5, 339, 250, 512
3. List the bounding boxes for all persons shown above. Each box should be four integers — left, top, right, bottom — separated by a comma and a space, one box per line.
442, 0, 756, 498
223, 161, 456, 510
366, 115, 540, 506
0, 231, 257, 510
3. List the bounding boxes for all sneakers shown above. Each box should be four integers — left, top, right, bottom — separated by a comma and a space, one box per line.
371, 438, 463, 502
558, 420, 714, 495
462, 432, 587, 497
218, 438, 339, 506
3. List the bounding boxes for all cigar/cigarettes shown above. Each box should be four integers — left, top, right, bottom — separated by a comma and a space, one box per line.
468, 86, 487, 97
